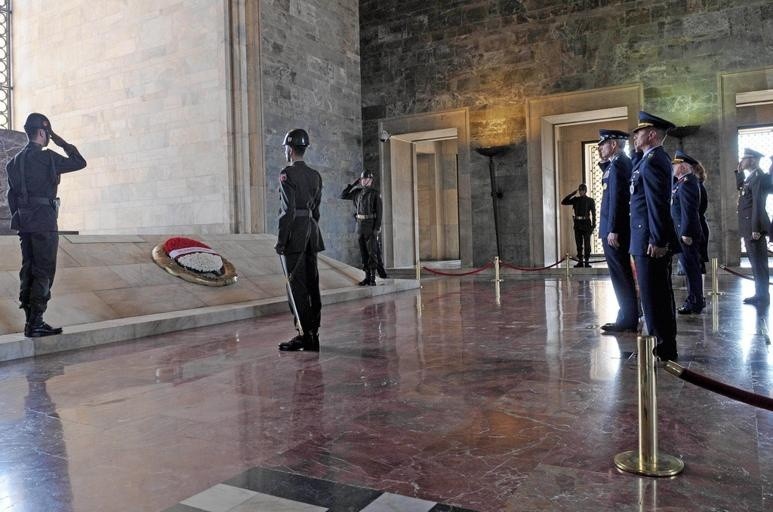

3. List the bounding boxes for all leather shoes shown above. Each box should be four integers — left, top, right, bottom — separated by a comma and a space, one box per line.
744, 296, 770, 305
678, 307, 701, 314
574, 263, 592, 268
26, 322, 62, 336
601, 323, 639, 332
359, 279, 376, 286
278, 335, 316, 351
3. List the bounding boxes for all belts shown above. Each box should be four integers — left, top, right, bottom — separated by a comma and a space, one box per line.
573, 216, 589, 220
295, 209, 313, 218
15, 197, 52, 206
355, 214, 376, 220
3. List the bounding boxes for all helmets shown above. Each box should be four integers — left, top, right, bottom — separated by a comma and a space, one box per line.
282, 129, 310, 146
360, 170, 374, 178
24, 113, 51, 130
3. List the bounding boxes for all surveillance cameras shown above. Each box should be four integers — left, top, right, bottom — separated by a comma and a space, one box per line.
379, 130, 389, 143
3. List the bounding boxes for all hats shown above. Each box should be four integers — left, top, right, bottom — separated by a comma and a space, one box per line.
671, 150, 699, 168
632, 110, 675, 134
740, 147, 764, 159
598, 128, 630, 145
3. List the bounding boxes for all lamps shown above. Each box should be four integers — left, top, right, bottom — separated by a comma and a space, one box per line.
667, 123, 701, 154
474, 145, 511, 263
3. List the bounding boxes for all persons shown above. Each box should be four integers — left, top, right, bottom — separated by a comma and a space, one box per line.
289, 353, 332, 479
677, 160, 709, 307
670, 150, 703, 313
341, 170, 382, 286
24, 366, 73, 512
628, 111, 683, 361
735, 148, 770, 304
274, 129, 325, 350
6, 113, 86, 336
561, 184, 595, 267
598, 129, 638, 332
